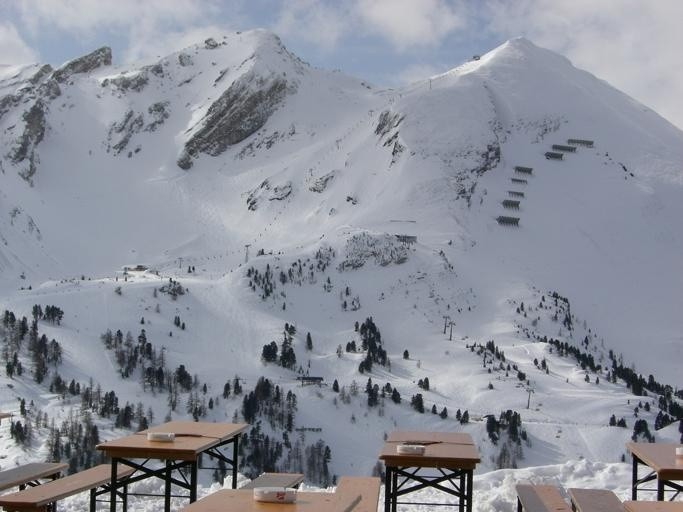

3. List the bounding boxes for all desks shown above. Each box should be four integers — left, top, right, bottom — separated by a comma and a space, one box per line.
95, 420, 252, 512
379, 431, 480, 512
626, 442, 683, 501
180, 487, 362, 512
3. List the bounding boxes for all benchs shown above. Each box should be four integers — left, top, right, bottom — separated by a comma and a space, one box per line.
624, 499, 683, 511
0, 463, 70, 492
239, 473, 304, 490
333, 476, 381, 512
1, 463, 137, 512
567, 488, 630, 512
515, 484, 572, 512
